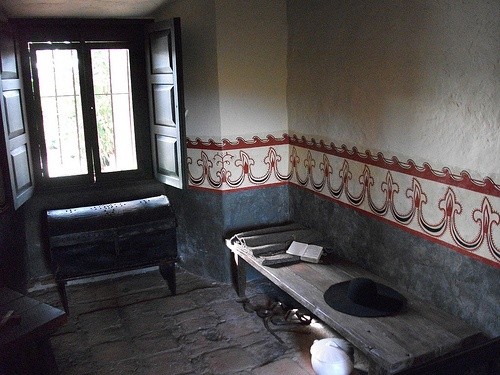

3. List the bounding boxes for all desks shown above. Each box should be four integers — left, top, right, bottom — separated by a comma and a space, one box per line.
0, 287, 66, 375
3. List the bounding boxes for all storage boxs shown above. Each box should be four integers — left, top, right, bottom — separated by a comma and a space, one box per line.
46, 195, 181, 315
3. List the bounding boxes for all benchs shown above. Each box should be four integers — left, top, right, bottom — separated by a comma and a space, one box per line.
225, 222, 484, 374
